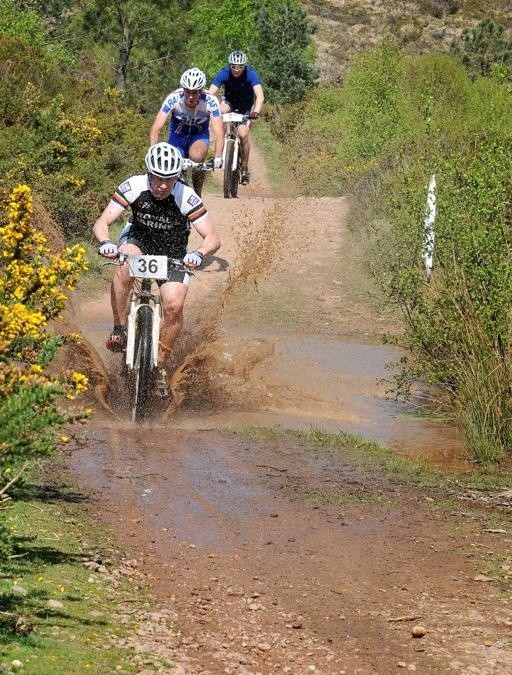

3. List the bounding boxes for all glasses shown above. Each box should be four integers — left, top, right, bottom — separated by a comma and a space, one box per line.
232, 66, 244, 70
184, 92, 199, 99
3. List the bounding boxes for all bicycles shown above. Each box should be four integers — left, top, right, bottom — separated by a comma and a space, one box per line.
96, 248, 205, 423
219, 112, 259, 198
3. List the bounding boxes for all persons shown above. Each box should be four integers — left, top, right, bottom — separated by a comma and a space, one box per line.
93, 142, 221, 399
207, 51, 265, 184
148, 67, 224, 201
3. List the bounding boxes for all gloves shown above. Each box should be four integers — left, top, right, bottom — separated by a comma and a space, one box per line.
182, 251, 204, 268
99, 240, 118, 258
248, 111, 257, 119
212, 157, 222, 171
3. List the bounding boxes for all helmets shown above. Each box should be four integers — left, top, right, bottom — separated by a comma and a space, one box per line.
180, 68, 206, 91
229, 51, 246, 64
145, 141, 183, 179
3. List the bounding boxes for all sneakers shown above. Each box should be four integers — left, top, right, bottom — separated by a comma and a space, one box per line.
240, 167, 249, 185
153, 366, 169, 400
106, 328, 127, 353
